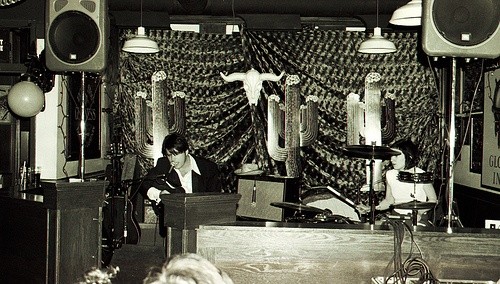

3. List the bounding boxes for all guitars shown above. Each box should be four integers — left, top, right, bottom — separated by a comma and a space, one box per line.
154, 178, 185, 207
103, 140, 141, 254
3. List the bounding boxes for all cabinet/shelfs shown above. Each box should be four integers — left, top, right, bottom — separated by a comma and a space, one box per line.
0, 19, 37, 184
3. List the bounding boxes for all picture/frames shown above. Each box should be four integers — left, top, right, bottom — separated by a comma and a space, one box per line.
65, 75, 101, 162
480, 65, 500, 193
469, 109, 483, 174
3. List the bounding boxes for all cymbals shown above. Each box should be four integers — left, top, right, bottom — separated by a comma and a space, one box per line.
270, 201, 327, 212
397, 171, 434, 184
394, 201, 437, 209
339, 145, 401, 156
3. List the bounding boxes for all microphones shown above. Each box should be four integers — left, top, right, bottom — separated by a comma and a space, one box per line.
144, 174, 167, 179
251, 185, 256, 208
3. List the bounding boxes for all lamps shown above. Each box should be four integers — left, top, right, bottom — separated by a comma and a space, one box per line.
388, 0, 423, 26
357, 1, 397, 54
122, 0, 159, 53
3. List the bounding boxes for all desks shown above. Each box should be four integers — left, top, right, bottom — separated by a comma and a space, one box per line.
0, 181, 110, 284
160, 193, 241, 259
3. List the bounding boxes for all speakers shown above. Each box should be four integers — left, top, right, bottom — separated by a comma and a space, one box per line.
234, 175, 300, 223
421, 0, 500, 63
45, 0, 111, 72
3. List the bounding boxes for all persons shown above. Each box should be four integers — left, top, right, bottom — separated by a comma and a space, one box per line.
374, 137, 437, 222
143, 252, 235, 283
138, 131, 222, 257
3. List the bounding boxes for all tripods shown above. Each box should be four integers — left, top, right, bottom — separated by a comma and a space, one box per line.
439, 58, 464, 229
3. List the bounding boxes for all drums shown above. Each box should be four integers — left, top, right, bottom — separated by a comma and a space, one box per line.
293, 187, 361, 224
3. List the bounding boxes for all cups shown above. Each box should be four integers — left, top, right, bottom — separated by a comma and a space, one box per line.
21, 167, 40, 191
